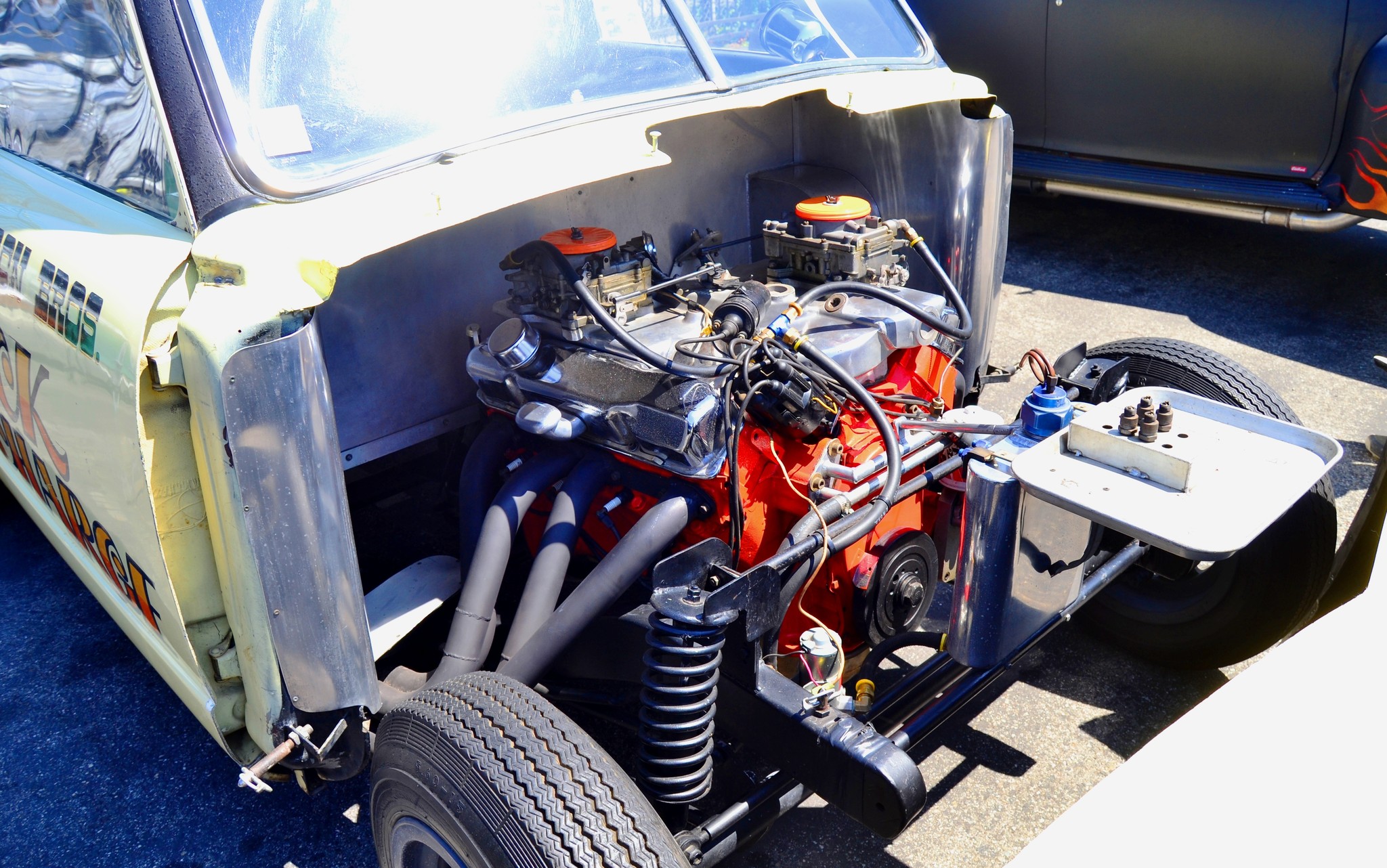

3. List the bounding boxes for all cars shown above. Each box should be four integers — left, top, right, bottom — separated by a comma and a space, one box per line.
0, 0, 1387, 868
